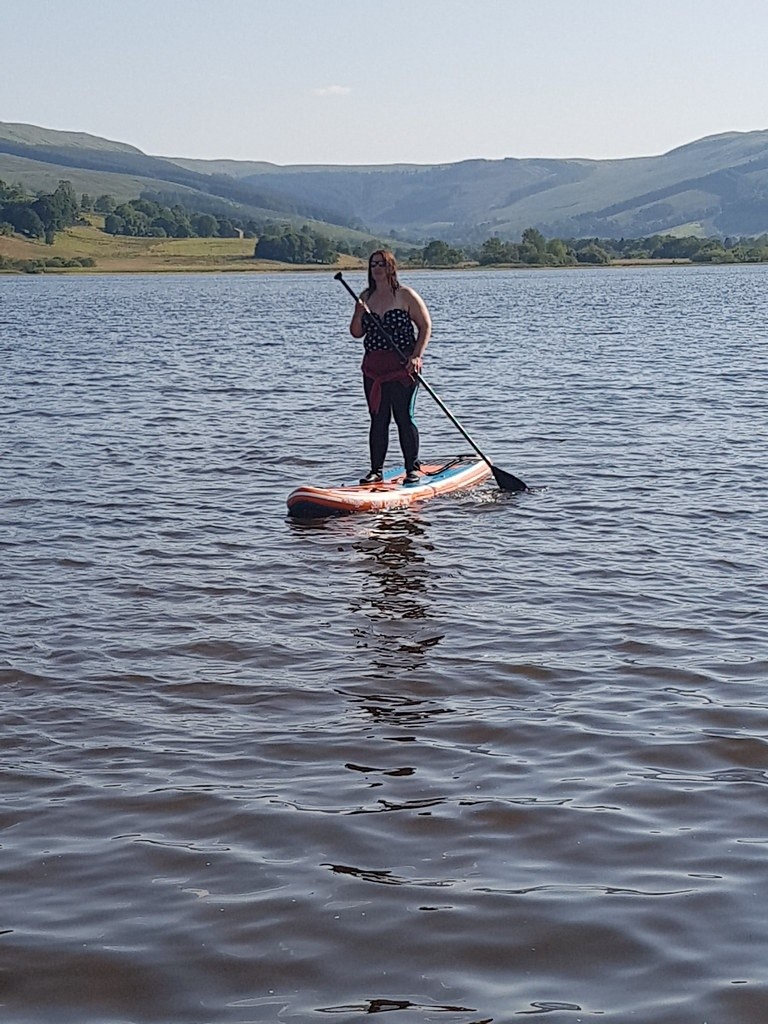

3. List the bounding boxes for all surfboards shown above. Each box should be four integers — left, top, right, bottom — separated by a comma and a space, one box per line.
284, 451, 494, 512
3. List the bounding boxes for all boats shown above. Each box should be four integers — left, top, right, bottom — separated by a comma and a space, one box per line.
286, 453, 493, 517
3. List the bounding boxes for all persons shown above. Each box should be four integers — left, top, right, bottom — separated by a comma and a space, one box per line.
349, 250, 432, 484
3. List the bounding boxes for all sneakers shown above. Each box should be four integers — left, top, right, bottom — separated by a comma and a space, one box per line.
403, 471, 420, 482
360, 470, 384, 485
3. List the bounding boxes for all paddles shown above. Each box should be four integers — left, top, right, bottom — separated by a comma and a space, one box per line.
330, 270, 532, 490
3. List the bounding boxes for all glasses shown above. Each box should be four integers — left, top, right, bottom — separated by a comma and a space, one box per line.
370, 260, 386, 267
